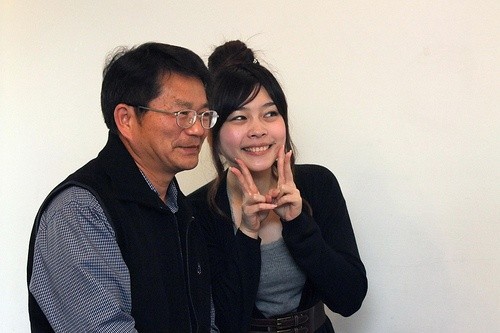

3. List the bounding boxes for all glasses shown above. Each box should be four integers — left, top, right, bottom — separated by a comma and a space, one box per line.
138, 105, 219, 130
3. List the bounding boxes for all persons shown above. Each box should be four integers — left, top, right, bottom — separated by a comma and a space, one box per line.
26, 41, 221, 333
185, 39, 369, 333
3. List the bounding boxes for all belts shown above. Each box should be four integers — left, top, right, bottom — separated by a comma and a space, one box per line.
248, 302, 327, 333
